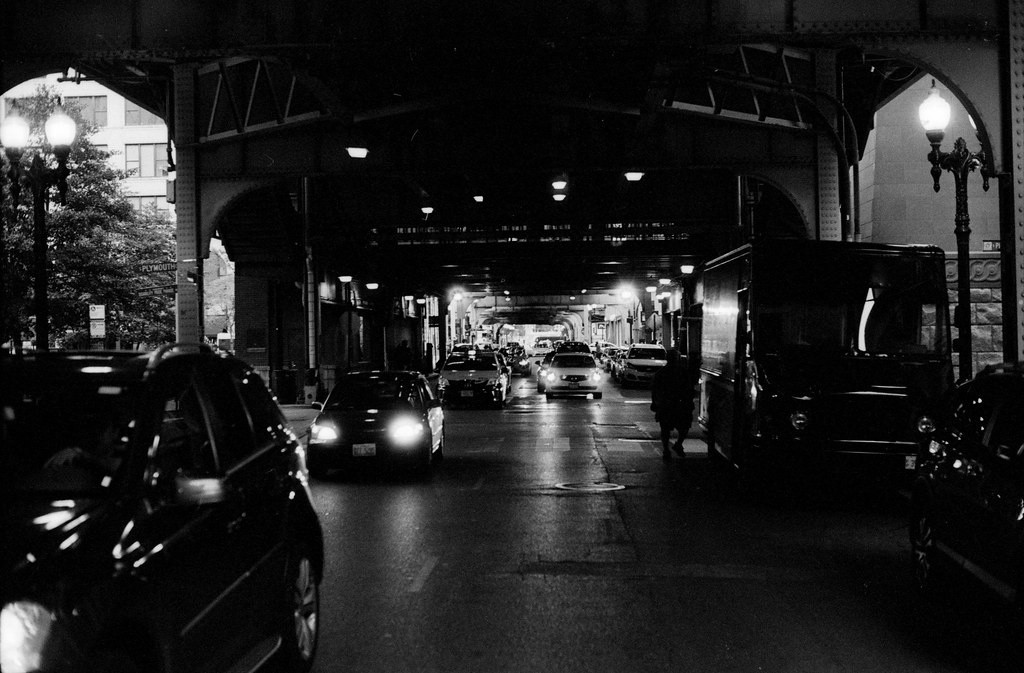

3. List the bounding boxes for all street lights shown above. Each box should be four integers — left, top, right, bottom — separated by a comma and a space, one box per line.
0, 104, 77, 351
916, 88, 995, 383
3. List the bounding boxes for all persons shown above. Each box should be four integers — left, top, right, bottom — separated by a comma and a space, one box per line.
651, 348, 695, 462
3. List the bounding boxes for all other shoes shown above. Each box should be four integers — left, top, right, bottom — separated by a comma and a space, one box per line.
663, 451, 672, 461
671, 443, 687, 458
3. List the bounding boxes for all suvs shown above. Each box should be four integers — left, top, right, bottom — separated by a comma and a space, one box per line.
0, 339, 327, 673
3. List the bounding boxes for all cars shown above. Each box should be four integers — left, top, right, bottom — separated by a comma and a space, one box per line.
306, 368, 446, 478
620, 342, 669, 390
900, 358, 1024, 644
543, 350, 607, 400
432, 350, 510, 410
437, 340, 629, 377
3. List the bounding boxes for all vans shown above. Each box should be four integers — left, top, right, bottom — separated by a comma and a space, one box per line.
698, 236, 969, 497
